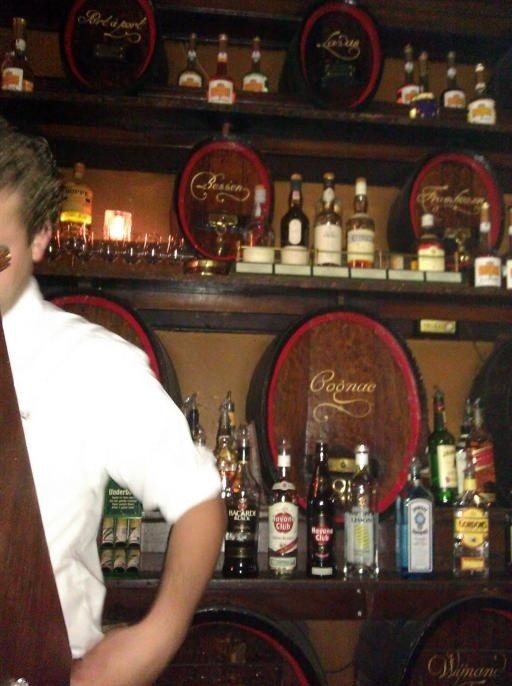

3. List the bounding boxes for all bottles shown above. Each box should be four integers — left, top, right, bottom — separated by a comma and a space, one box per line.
314, 174, 342, 267
409, 52, 439, 119
429, 393, 457, 506
225, 428, 257, 578
181, 394, 206, 446
345, 177, 376, 268
1, 18, 39, 98
420, 213, 445, 273
467, 401, 495, 506
176, 36, 210, 87
238, 185, 274, 264
205, 33, 236, 104
474, 202, 502, 287
451, 469, 491, 578
457, 398, 471, 500
396, 457, 433, 577
440, 52, 467, 107
467, 64, 497, 124
507, 226, 512, 288
281, 174, 308, 265
396, 45, 421, 103
346, 445, 377, 579
269, 439, 296, 578
60, 161, 93, 245
215, 398, 239, 484
307, 443, 336, 578
243, 37, 269, 93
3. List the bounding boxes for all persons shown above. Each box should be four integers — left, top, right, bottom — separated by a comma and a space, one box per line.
1, 130, 226, 686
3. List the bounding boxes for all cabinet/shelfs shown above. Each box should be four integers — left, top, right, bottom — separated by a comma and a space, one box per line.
0, 0, 512, 619
